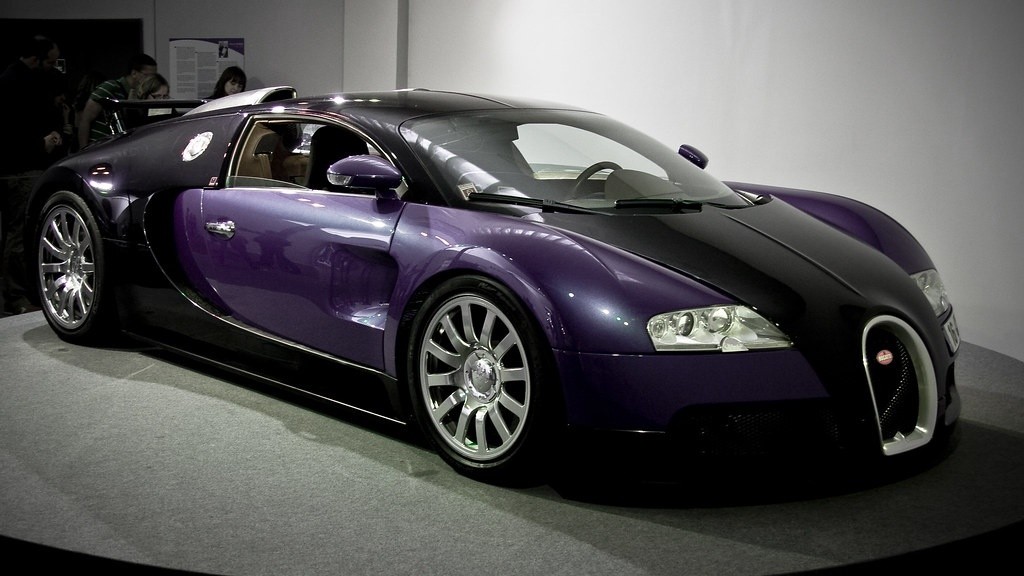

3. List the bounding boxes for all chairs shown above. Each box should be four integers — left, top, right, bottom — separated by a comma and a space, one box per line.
246, 154, 308, 186
301, 125, 371, 192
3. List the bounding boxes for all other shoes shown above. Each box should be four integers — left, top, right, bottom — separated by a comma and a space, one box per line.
4, 298, 41, 315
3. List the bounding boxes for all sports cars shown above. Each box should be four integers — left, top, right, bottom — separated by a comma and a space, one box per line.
20, 85, 961, 478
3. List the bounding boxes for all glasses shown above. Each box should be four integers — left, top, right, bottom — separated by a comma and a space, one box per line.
150, 94, 169, 100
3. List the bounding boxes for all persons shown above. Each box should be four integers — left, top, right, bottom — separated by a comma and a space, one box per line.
0, 34, 178, 315
197, 66, 246, 100
